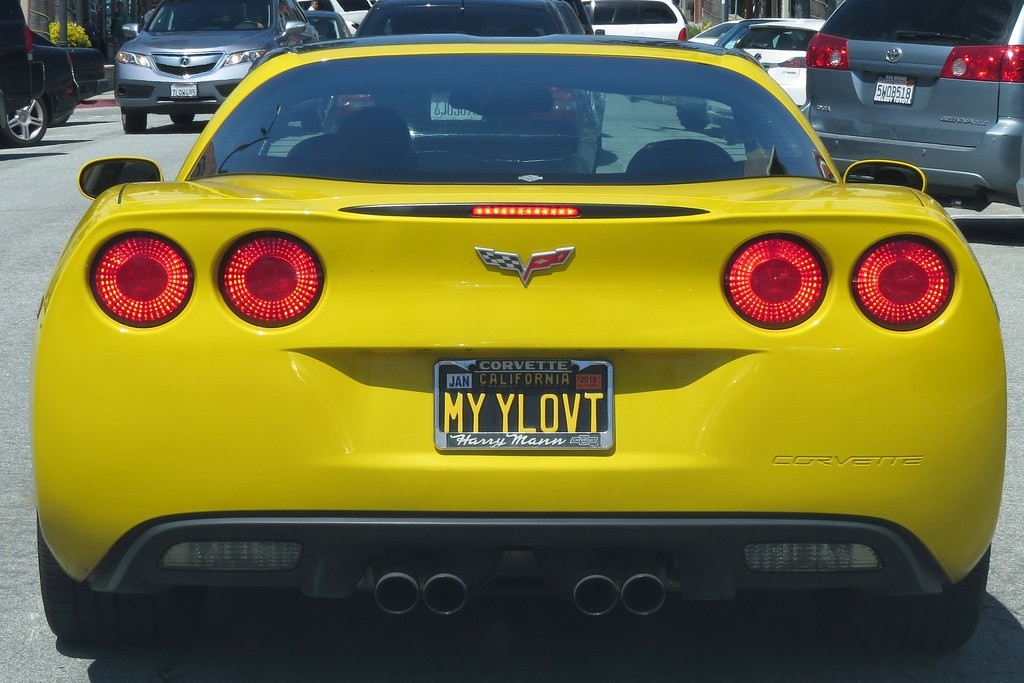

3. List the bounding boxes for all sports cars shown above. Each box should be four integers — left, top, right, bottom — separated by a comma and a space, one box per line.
33, 31, 1005, 652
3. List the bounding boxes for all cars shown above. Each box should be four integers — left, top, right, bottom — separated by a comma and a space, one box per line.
296, 1, 378, 38
115, 0, 319, 132
0, 31, 109, 148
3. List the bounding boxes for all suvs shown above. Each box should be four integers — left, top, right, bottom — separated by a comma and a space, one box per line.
582, 1, 689, 44
0, 0, 46, 132
707, 21, 827, 135
326, 1, 605, 179
800, 0, 1024, 213
627, 20, 790, 130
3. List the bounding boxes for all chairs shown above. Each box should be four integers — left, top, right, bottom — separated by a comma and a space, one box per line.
624, 138, 738, 184
288, 134, 406, 185
